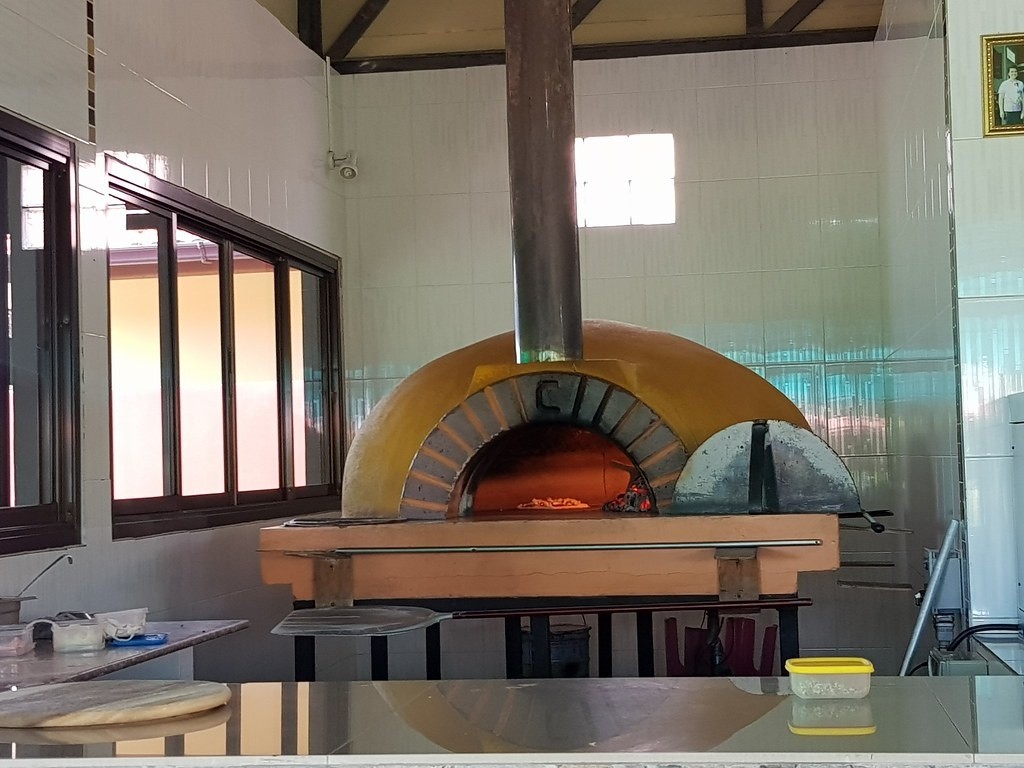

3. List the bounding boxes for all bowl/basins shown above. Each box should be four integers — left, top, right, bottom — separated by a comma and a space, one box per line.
784, 657, 875, 699
0, 624, 34, 657
51, 618, 105, 652
94, 607, 148, 639
787, 698, 877, 736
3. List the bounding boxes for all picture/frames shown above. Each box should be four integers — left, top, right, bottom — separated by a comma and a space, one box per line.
980, 32, 1024, 136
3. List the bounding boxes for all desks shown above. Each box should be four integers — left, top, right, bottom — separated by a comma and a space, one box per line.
1, 670, 1023, 754
0, 619, 253, 691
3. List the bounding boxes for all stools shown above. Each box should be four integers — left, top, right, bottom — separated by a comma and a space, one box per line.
660, 613, 778, 675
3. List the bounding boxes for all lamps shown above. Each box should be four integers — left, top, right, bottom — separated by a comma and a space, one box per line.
328, 148, 360, 179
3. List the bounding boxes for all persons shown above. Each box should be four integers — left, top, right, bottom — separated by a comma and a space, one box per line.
998, 66, 1024, 126
